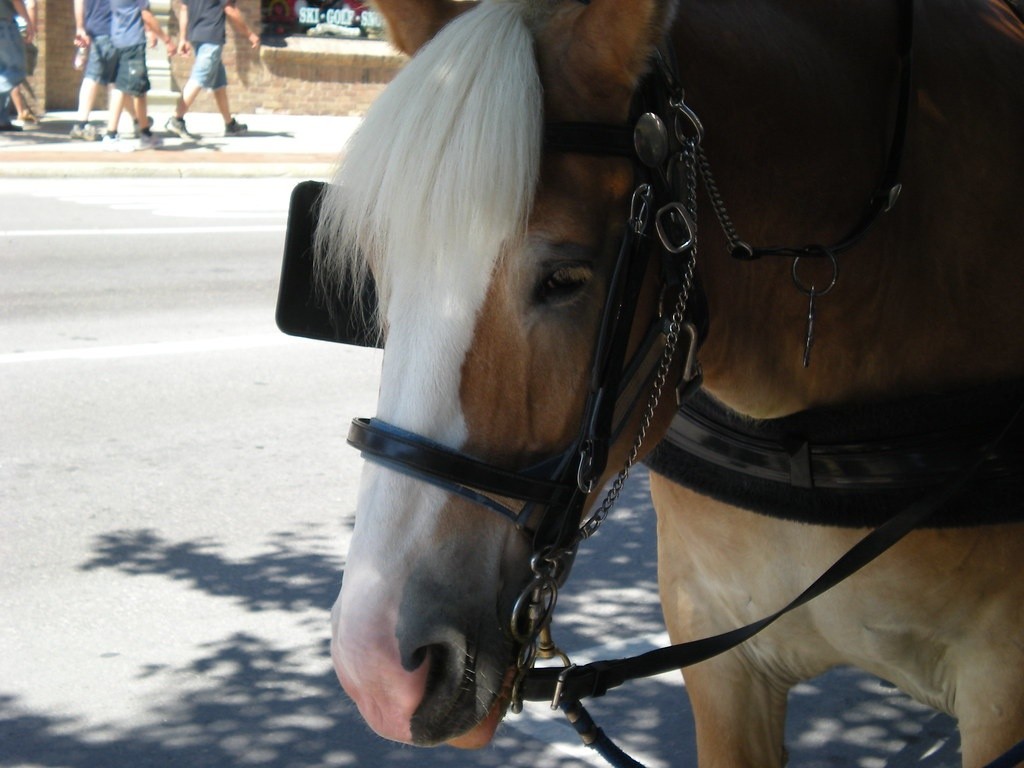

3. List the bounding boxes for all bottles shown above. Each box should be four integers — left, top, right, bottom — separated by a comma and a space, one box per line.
74, 41, 88, 71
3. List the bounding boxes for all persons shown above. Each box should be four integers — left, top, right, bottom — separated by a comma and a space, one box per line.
0, 0, 260, 143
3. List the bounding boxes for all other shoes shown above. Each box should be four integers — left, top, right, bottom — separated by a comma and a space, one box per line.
1, 120, 23, 133
224, 121, 249, 138
16, 108, 40, 125
166, 117, 195, 141
134, 133, 164, 149
71, 124, 104, 142
101, 134, 135, 153
132, 116, 155, 135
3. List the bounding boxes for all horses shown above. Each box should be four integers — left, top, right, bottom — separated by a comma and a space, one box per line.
312, 0, 1024, 765
